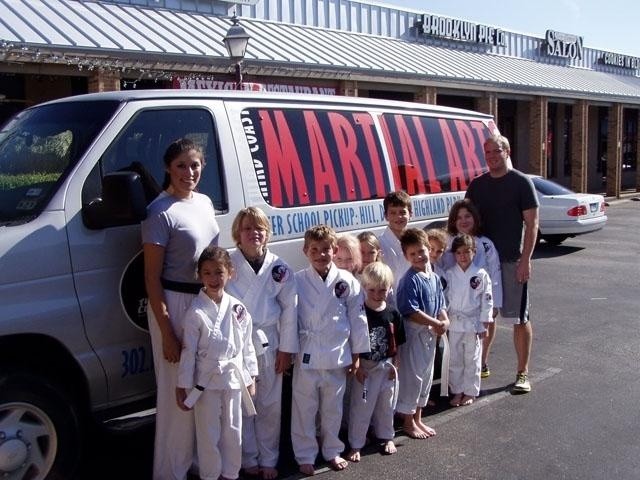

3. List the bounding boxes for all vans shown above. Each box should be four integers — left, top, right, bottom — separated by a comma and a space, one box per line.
0, 89, 514, 480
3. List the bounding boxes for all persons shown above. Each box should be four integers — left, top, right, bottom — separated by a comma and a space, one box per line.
225, 189, 504, 480
464, 134, 539, 395
140, 137, 221, 480
174, 245, 259, 480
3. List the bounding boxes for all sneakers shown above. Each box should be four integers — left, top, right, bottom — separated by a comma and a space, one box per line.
513, 372, 531, 393
481, 363, 490, 378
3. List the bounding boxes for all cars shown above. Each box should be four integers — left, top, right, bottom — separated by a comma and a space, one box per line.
517, 172, 609, 244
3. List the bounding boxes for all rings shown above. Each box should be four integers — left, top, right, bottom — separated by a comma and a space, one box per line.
518, 280, 522, 282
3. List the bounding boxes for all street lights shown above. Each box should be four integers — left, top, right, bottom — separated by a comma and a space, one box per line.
222, 15, 252, 89
